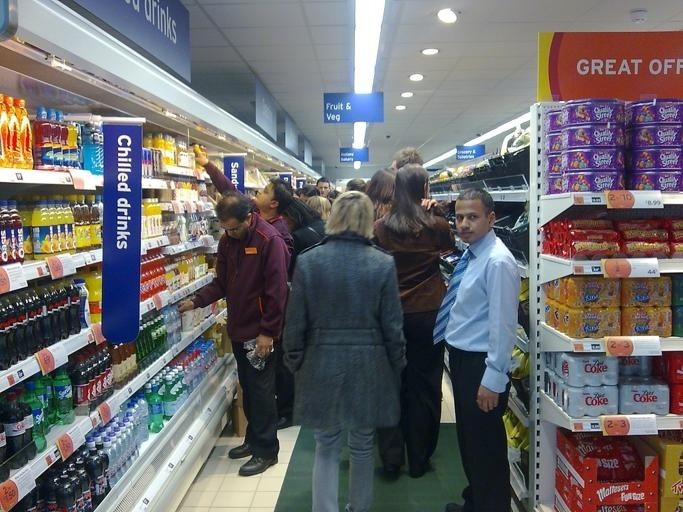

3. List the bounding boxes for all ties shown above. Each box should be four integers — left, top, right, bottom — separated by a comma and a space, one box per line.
432, 250, 471, 345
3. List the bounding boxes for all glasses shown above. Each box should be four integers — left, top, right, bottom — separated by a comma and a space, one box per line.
222, 224, 243, 234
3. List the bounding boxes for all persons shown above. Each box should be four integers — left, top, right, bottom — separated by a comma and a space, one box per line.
435, 187, 520, 511
176, 193, 289, 477
280, 189, 409, 512
374, 163, 455, 477
194, 146, 425, 431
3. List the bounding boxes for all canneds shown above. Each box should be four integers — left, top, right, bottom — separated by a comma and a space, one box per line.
546, 352, 671, 417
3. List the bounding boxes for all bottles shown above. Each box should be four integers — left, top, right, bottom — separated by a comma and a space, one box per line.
0, 94, 207, 179
0, 304, 181, 483
0, 195, 162, 266
0, 247, 206, 369
15, 336, 218, 512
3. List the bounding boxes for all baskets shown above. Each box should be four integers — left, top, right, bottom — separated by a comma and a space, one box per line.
492, 211, 528, 265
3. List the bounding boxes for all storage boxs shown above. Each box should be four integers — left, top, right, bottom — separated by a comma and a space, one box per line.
555, 429, 659, 512
640, 435, 683, 512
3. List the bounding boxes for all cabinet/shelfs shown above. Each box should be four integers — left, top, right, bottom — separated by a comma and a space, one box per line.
426, 146, 535, 512
0, 166, 240, 512
539, 190, 683, 512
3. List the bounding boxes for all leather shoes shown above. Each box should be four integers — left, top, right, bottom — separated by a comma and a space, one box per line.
238, 455, 278, 476
446, 503, 463, 512
410, 459, 430, 479
384, 464, 400, 477
228, 443, 253, 459
277, 415, 294, 430
